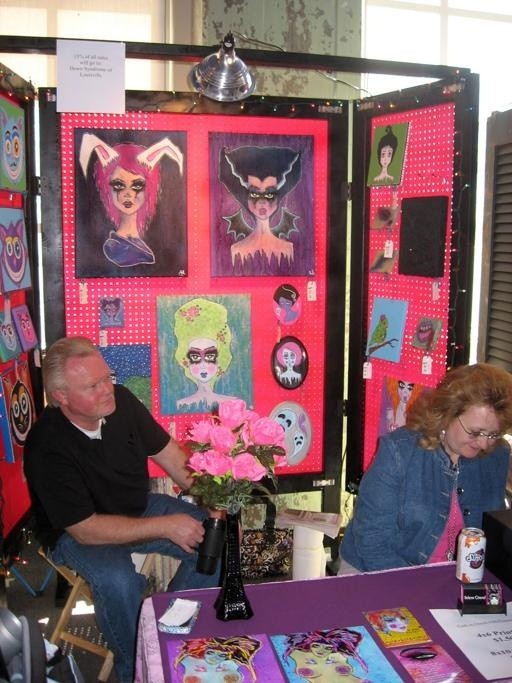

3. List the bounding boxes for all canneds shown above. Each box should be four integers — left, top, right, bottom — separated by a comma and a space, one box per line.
456, 527, 487, 583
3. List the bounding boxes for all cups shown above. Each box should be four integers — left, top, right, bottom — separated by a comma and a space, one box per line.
192, 517, 227, 575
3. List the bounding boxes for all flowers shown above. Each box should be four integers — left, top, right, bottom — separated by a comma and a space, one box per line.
183, 396, 288, 516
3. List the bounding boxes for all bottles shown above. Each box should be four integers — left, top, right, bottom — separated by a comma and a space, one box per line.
196, 520, 223, 576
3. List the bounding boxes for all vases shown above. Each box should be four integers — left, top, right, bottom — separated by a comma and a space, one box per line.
213, 512, 253, 621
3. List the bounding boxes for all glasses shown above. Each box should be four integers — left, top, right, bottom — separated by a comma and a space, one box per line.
457, 417, 506, 439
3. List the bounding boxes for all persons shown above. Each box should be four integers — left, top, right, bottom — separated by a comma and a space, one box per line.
281, 628, 373, 683
338, 362, 512, 575
173, 298, 238, 412
373, 126, 398, 182
24, 337, 225, 683
79, 132, 183, 268
218, 144, 301, 272
275, 342, 302, 386
173, 635, 264, 683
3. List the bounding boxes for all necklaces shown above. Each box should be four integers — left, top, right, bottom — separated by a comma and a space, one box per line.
445, 459, 460, 563
